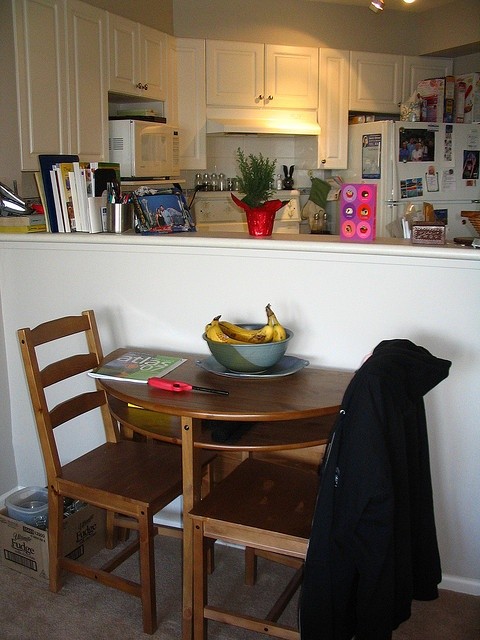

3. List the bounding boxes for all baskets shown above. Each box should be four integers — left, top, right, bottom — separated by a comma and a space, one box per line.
460, 211, 480, 234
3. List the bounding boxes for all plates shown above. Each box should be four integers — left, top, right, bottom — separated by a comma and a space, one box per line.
195, 355, 311, 379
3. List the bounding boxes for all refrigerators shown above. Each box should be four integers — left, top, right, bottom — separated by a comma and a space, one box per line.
347, 120, 480, 239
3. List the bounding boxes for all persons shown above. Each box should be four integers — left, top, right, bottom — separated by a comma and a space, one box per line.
160, 205, 173, 226
155, 208, 166, 225
400, 137, 435, 161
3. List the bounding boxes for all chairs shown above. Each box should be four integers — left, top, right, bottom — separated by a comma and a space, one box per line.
15, 310, 222, 635
185, 337, 430, 639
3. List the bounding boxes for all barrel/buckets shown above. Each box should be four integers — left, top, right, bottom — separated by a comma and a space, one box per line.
4, 485, 48, 527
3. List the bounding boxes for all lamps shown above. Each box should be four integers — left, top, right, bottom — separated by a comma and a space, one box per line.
369, 0, 386, 15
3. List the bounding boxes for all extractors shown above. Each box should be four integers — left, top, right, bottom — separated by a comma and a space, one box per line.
205, 110, 322, 136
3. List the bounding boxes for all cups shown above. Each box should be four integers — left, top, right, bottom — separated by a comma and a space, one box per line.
106, 201, 135, 234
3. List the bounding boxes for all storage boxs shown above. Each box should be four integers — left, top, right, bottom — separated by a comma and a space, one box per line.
0, 481, 114, 586
410, 215, 446, 245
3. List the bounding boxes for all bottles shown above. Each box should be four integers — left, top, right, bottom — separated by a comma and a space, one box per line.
203, 173, 212, 191
218, 173, 226, 191
194, 174, 204, 192
276, 173, 283, 190
271, 174, 278, 189
212, 173, 220, 191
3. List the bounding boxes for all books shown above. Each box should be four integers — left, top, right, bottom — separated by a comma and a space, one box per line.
0, 215, 46, 233
87, 351, 188, 383
34, 155, 121, 233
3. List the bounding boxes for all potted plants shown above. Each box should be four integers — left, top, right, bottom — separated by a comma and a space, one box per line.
228, 146, 290, 236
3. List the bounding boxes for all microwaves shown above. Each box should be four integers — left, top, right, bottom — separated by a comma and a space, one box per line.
108, 118, 183, 178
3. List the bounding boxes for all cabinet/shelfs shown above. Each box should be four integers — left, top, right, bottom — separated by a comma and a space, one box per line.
161, 32, 208, 172
348, 50, 455, 113
315, 47, 351, 172
0, 0, 112, 173
108, 10, 168, 104
202, 37, 319, 117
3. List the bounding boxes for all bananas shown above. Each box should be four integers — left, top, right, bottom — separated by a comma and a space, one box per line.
205, 315, 256, 345
219, 303, 286, 344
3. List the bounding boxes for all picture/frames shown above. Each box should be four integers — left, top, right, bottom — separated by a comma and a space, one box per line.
127, 187, 197, 235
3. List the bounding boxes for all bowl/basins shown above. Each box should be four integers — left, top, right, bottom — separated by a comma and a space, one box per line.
202, 324, 294, 374
